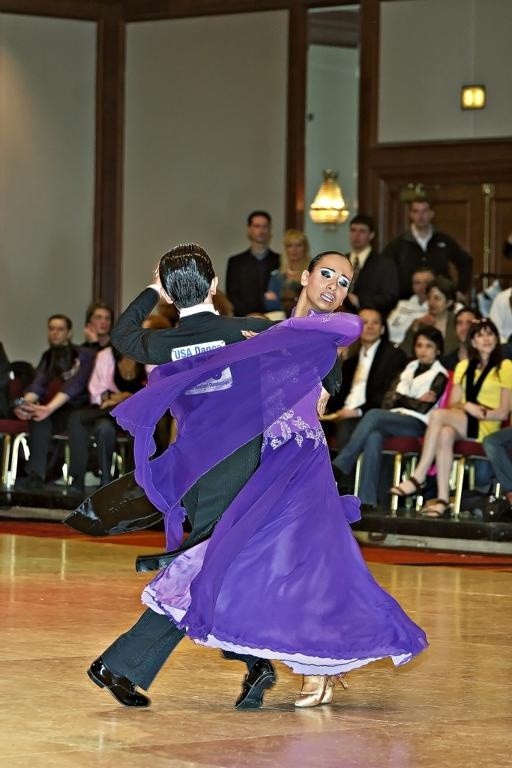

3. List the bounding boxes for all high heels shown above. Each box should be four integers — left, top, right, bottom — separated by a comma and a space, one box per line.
293, 674, 329, 707
321, 675, 348, 704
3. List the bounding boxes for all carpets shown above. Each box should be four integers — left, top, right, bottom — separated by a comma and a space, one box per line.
2, 506, 512, 575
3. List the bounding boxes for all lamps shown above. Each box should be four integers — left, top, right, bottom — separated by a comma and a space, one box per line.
306, 166, 353, 229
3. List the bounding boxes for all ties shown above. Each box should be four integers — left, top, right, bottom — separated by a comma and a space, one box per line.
349, 256, 359, 291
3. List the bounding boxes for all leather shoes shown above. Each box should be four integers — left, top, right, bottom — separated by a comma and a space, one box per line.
484, 494, 512, 521
235, 657, 277, 709
67, 486, 86, 498
461, 483, 494, 509
14, 473, 45, 494
87, 658, 152, 708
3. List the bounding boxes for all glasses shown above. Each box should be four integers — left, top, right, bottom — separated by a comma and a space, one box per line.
469, 317, 490, 326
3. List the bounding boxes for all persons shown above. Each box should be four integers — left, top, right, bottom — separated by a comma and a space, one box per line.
107, 252, 429, 708
62, 244, 345, 710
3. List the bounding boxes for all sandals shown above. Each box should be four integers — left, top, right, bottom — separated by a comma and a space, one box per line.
386, 476, 430, 497
423, 499, 454, 518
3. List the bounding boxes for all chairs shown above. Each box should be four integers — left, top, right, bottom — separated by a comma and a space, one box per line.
0, 335, 171, 505
316, 286, 512, 532
